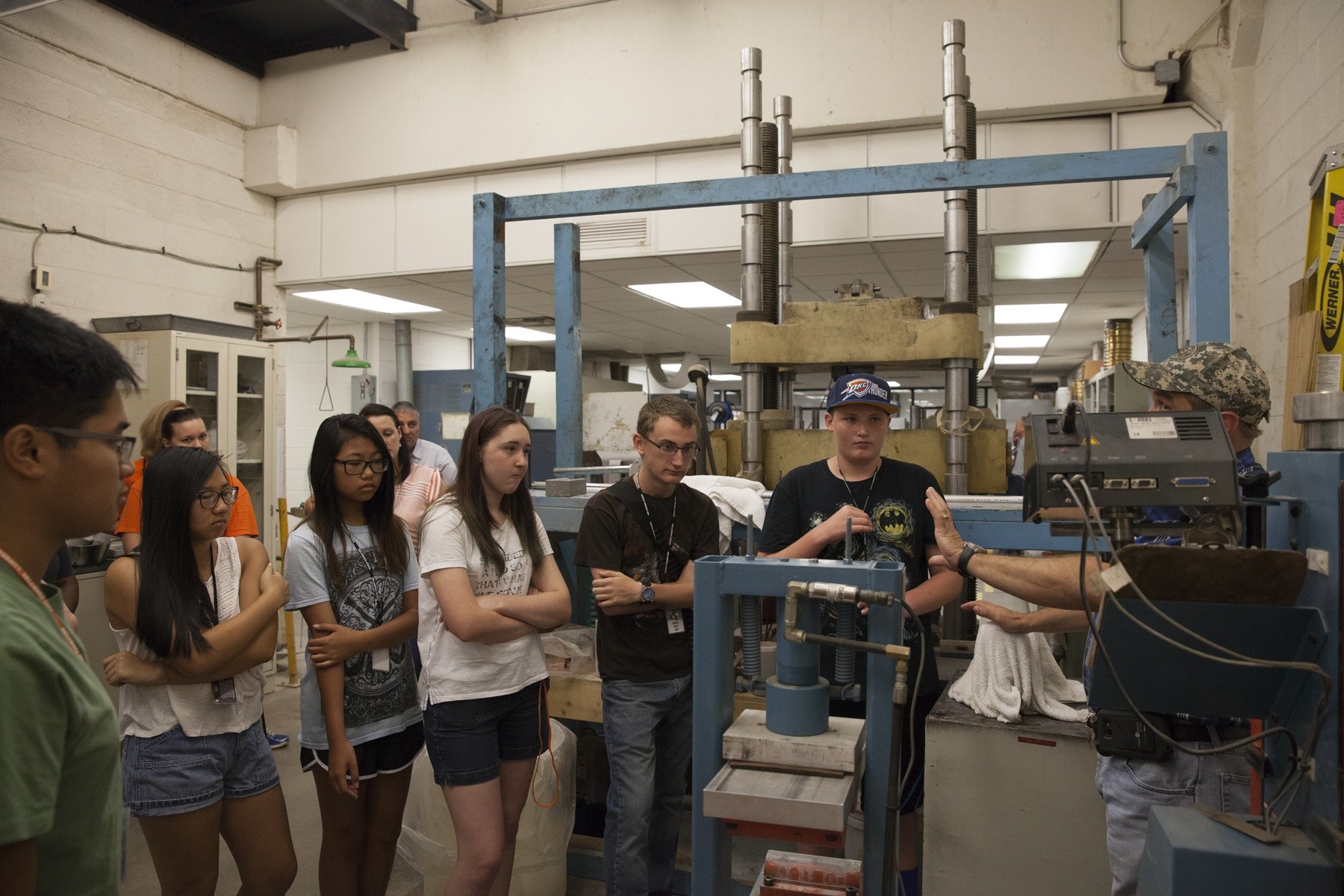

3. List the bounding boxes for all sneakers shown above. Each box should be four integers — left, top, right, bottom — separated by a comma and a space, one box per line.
265, 728, 290, 749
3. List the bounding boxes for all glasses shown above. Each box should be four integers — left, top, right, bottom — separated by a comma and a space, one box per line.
193, 486, 239, 509
637, 431, 700, 458
34, 424, 136, 463
333, 459, 389, 475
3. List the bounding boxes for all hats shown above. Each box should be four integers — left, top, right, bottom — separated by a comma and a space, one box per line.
1122, 341, 1271, 426
826, 373, 899, 414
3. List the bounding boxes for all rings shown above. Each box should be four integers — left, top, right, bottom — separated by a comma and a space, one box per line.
942, 515, 949, 519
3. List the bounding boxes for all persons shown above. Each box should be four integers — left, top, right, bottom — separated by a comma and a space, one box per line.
570, 394, 721, 896
0, 300, 142, 896
925, 339, 1275, 896
104, 445, 299, 896
419, 406, 571, 896
391, 400, 464, 486
119, 400, 290, 748
1006, 418, 1027, 497
283, 414, 422, 896
756, 373, 974, 896
303, 402, 448, 562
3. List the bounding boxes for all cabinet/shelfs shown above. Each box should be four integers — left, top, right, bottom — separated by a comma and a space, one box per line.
96, 329, 278, 574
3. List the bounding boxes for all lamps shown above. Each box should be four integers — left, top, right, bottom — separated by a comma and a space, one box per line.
259, 334, 370, 368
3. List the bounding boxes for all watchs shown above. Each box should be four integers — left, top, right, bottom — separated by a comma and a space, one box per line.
957, 541, 987, 579
641, 582, 655, 611
